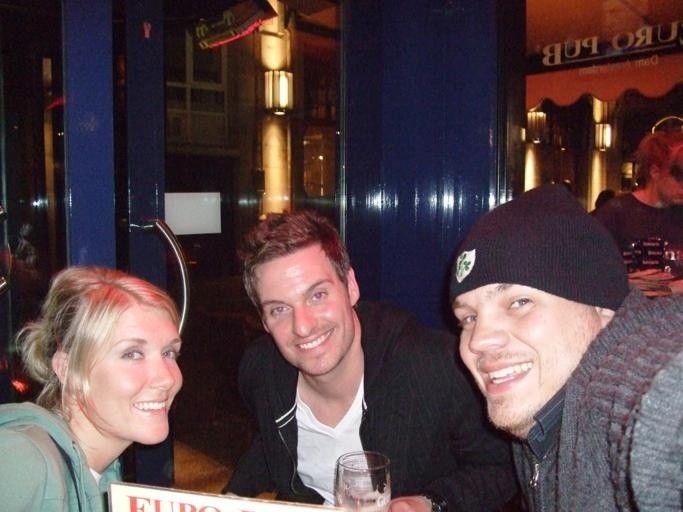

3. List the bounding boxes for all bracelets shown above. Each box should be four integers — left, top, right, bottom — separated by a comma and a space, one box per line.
418, 489, 448, 512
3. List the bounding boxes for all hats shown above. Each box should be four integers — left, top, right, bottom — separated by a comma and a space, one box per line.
448, 181, 628, 311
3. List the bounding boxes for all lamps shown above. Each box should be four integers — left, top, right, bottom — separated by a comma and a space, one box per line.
264, 71, 295, 116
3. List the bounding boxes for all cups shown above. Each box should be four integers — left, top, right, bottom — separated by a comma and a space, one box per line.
335, 451, 391, 512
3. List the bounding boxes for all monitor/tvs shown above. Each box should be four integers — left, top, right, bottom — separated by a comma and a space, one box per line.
164, 192, 224, 236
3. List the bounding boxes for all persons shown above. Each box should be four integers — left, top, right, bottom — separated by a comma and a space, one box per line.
595, 123, 683, 248
447, 180, 683, 512
0, 262, 186, 512
219, 209, 519, 512
